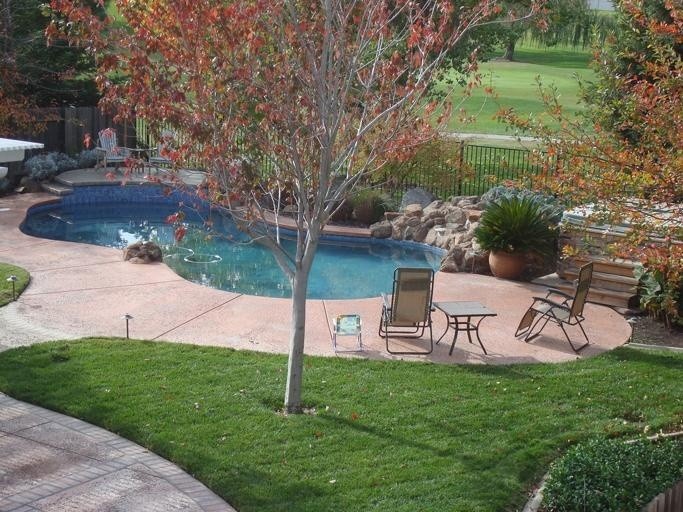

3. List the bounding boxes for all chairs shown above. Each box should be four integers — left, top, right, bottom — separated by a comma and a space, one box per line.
333, 261, 594, 355
96, 128, 177, 175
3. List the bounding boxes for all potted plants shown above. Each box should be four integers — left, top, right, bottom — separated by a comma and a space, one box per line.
473, 191, 557, 280
